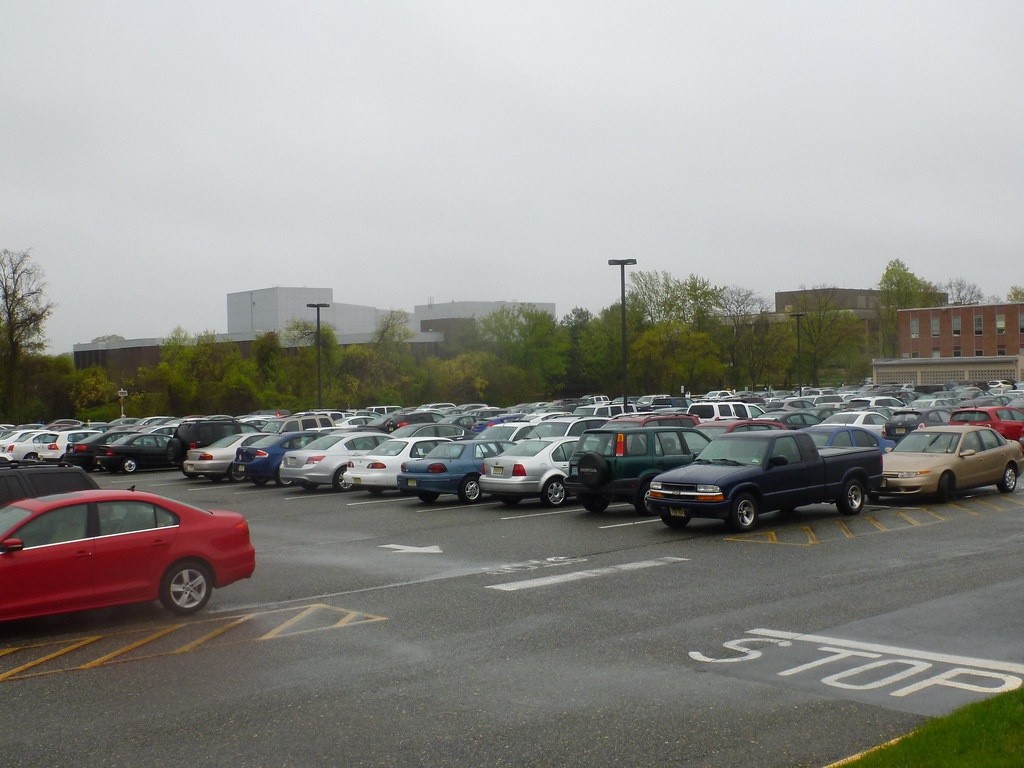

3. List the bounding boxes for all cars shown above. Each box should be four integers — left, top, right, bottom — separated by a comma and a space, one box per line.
868, 425, 1024, 507
477, 435, 617, 507
398, 444, 539, 503
37, 428, 107, 466
278, 431, 404, 491
232, 430, 340, 488
341, 438, 484, 498
1, 461, 117, 539
65, 432, 154, 476
0, 380, 1024, 471
92, 432, 174, 474
182, 433, 277, 482
0, 488, 257, 623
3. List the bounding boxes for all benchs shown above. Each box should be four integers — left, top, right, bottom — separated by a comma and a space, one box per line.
115, 509, 169, 532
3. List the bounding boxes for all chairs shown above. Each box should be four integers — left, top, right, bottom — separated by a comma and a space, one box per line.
661, 438, 678, 454
631, 438, 644, 453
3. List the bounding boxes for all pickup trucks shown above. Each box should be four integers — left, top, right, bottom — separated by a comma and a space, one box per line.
646, 431, 883, 531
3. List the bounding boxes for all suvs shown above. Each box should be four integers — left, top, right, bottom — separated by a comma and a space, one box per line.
685, 401, 764, 421
563, 427, 716, 515
168, 419, 267, 479
261, 415, 335, 433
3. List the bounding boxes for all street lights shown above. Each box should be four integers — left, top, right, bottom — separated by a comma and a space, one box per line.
608, 260, 639, 411
791, 313, 804, 397
308, 302, 330, 409
